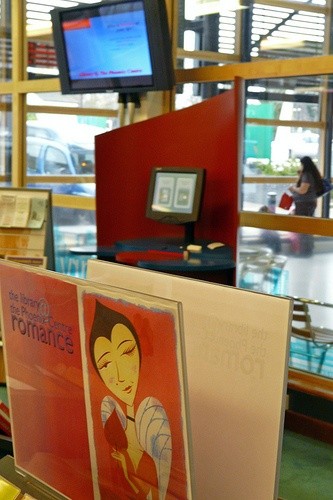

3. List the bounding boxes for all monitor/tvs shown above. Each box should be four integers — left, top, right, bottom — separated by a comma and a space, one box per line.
52, 2, 177, 97
146, 167, 207, 251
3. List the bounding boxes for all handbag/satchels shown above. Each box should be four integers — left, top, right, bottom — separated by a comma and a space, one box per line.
278, 186, 295, 210
316, 174, 333, 199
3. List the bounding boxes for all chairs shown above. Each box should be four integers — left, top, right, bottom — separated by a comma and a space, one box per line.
286, 295, 333, 376
237, 245, 288, 294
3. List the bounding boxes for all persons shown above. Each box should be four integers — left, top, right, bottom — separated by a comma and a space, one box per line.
288, 156, 321, 257
258, 206, 282, 254
